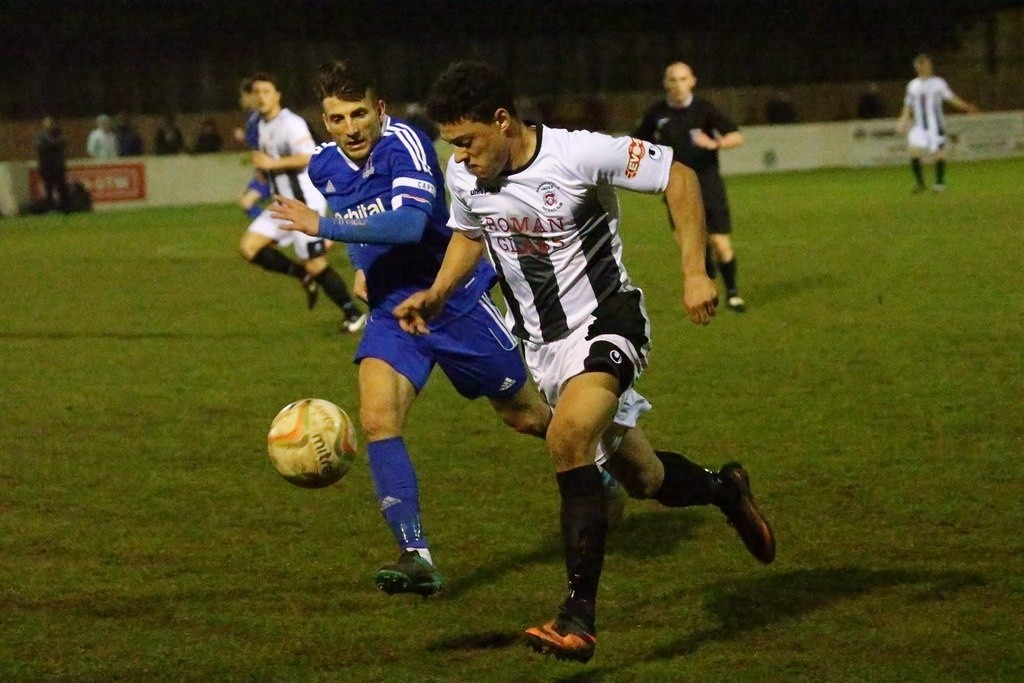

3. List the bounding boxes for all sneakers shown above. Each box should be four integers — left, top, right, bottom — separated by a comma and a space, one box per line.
523, 605, 597, 664
718, 460, 776, 564
376, 547, 443, 598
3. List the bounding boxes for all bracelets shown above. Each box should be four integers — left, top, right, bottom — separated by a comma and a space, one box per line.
713, 138, 721, 149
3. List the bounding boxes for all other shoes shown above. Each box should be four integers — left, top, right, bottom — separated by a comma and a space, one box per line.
600, 468, 624, 532
299, 273, 318, 311
341, 312, 365, 332
933, 183, 946, 194
726, 291, 744, 311
913, 182, 927, 194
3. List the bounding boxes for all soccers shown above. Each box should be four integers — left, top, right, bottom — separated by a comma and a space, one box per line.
266, 398, 355, 492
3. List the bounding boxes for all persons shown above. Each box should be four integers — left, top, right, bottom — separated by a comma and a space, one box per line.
858, 82, 886, 119
87, 114, 119, 158
894, 53, 974, 195
235, 73, 367, 334
155, 112, 182, 155
33, 115, 69, 198
114, 112, 144, 157
390, 56, 775, 662
631, 61, 748, 312
270, 60, 627, 593
192, 117, 225, 153
